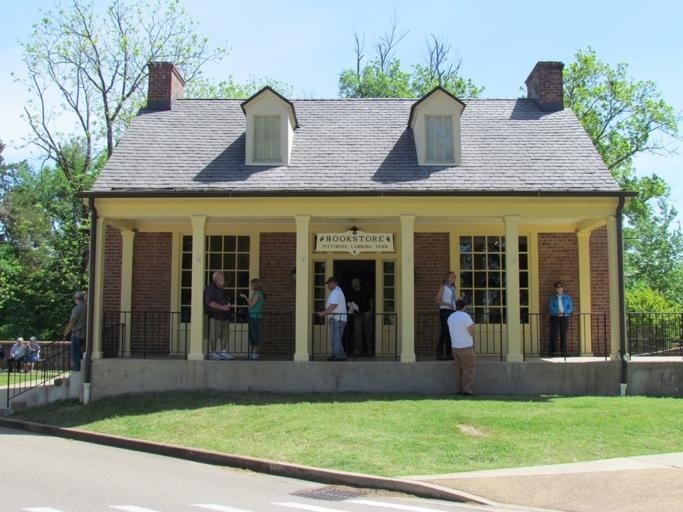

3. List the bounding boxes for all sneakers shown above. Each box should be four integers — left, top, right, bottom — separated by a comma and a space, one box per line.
221, 352, 234, 360
437, 356, 454, 361
326, 355, 345, 361
250, 354, 259, 360
210, 353, 223, 359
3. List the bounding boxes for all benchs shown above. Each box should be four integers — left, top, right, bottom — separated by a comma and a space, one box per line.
3, 357, 46, 372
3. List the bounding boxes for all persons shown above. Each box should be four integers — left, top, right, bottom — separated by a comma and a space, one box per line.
205, 272, 235, 360
240, 279, 266, 360
548, 282, 573, 357
63, 292, 87, 371
447, 300, 477, 396
348, 278, 375, 357
23, 336, 41, 373
6, 337, 26, 373
318, 277, 348, 361
435, 272, 457, 361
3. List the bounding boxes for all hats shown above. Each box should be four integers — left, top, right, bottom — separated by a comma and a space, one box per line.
73, 292, 84, 299
325, 277, 336, 283
